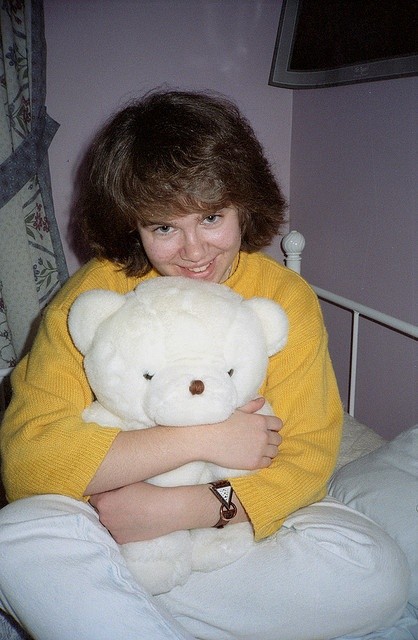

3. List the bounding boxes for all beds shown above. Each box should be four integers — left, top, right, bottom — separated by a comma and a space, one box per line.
0, 231, 418, 640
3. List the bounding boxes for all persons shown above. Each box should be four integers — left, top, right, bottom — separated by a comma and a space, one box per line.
1, 88, 409, 640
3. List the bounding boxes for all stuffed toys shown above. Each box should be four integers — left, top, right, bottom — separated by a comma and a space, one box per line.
68, 276, 288, 595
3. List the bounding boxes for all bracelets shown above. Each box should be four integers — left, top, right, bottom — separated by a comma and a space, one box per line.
207, 480, 237, 528
